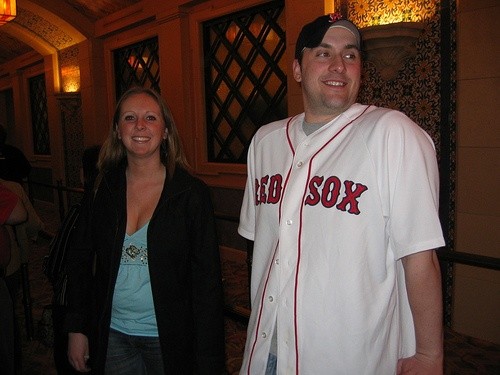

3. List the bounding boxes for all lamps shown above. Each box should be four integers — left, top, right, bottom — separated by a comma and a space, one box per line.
54, 92, 80, 117
0, 0, 17, 28
358, 21, 427, 80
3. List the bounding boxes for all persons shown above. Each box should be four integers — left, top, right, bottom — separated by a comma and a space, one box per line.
0, 124, 103, 374
63, 86, 232, 375
237, 13, 448, 375
